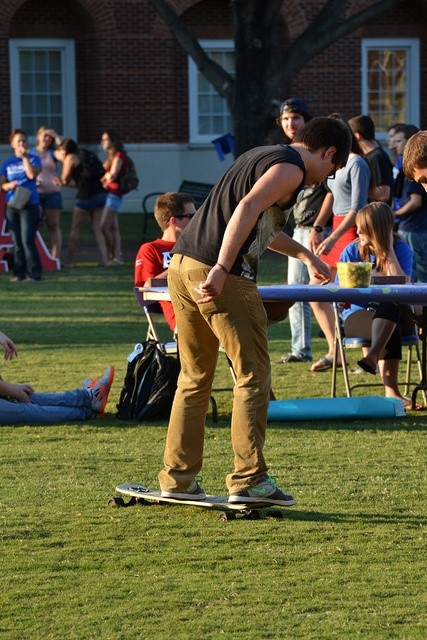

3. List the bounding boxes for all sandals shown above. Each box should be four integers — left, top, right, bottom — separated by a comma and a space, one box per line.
105, 258, 124, 266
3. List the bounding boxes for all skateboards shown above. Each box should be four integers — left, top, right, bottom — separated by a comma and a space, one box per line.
109, 484, 283, 522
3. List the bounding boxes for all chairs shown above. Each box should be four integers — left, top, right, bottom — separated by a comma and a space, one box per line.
332, 302, 425, 407
144, 305, 236, 424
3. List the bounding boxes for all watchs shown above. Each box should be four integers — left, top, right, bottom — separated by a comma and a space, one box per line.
310, 221, 326, 236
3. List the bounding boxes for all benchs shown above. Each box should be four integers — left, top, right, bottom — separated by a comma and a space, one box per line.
142, 180, 216, 232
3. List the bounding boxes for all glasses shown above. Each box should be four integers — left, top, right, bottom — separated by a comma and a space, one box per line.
167, 213, 194, 222
327, 158, 337, 179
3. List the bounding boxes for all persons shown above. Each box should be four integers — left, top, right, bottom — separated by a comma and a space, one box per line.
97, 126, 127, 270
396, 131, 426, 196
394, 125, 427, 282
336, 201, 416, 409
308, 112, 368, 383
278, 99, 332, 364
156, 116, 352, 507
31, 128, 67, 274
381, 121, 403, 150
344, 114, 389, 203
0, 126, 41, 285
54, 137, 112, 270
0, 367, 118, 423
135, 190, 297, 341
1, 324, 18, 363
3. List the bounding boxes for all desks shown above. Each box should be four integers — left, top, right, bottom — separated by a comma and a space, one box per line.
139, 285, 426, 423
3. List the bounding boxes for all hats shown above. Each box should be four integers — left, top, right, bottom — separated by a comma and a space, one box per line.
279, 98, 310, 113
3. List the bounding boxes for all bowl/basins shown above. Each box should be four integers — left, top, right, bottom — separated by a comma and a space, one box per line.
337, 261, 373, 288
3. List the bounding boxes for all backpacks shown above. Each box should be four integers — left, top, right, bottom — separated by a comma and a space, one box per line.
116, 339, 182, 422
117, 151, 139, 195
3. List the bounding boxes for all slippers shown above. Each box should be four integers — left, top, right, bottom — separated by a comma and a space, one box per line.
309, 356, 349, 373
357, 358, 376, 375
274, 353, 312, 364
403, 397, 423, 411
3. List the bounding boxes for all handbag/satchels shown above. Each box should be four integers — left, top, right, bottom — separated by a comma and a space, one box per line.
8, 187, 32, 210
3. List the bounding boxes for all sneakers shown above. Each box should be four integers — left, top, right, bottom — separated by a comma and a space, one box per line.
10, 276, 20, 282
23, 277, 35, 281
84, 378, 105, 419
90, 366, 115, 412
162, 481, 206, 500
227, 475, 295, 506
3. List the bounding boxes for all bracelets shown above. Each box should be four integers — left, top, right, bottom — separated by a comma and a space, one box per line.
214, 259, 236, 279
